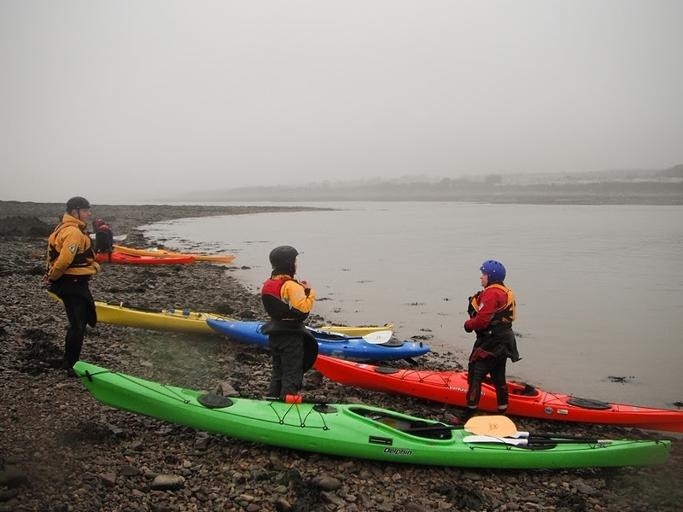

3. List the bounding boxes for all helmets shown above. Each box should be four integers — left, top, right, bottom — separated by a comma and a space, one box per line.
66, 198, 90, 211
270, 246, 298, 268
480, 260, 506, 286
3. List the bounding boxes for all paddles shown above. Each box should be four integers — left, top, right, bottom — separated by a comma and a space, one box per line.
324, 330, 392, 344
462, 435, 598, 445
396, 415, 518, 437
505, 432, 598, 440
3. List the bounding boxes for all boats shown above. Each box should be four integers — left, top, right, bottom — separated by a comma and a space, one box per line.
73, 360, 671, 467
96, 252, 195, 265
115, 245, 236, 263
206, 317, 431, 361
45, 290, 396, 338
313, 354, 683, 433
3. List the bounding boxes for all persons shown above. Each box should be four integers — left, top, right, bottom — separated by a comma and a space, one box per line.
40, 197, 101, 377
94, 224, 113, 264
460, 259, 516, 420
262, 245, 314, 402
93, 216, 104, 231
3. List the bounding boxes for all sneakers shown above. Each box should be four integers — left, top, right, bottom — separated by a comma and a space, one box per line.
67, 368, 78, 377
449, 415, 473, 424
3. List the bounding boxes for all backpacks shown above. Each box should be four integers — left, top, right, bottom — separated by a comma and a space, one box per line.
468, 283, 517, 325
